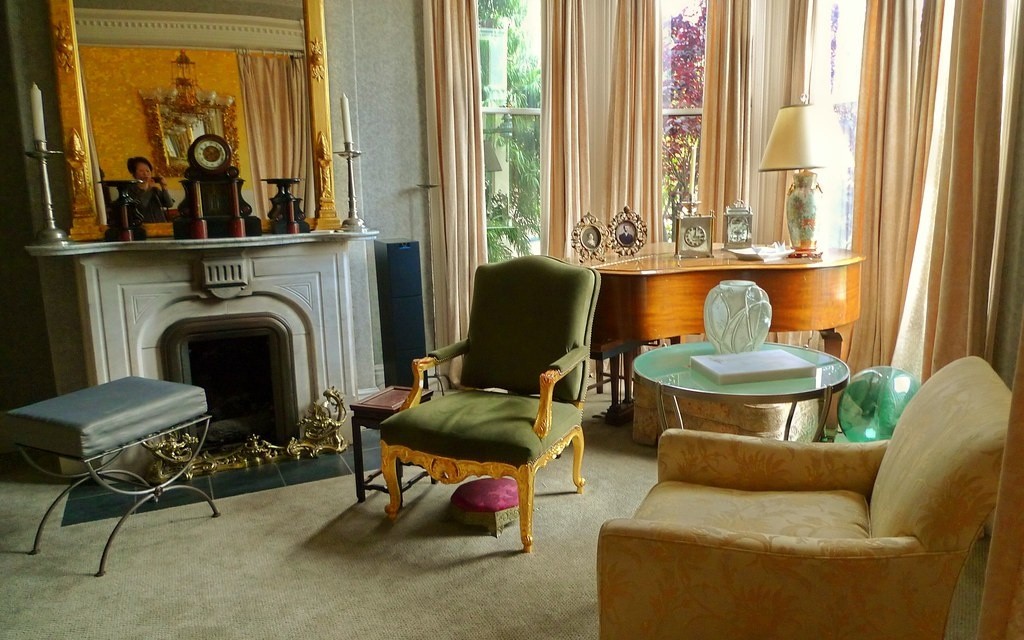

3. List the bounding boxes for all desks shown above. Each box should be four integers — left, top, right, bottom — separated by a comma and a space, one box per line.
630, 341, 850, 443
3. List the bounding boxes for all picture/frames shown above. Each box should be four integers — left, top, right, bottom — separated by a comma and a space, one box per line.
570, 205, 647, 267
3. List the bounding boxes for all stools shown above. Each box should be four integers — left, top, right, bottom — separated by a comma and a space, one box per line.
349, 386, 434, 511
0, 376, 221, 578
451, 477, 520, 539
632, 380, 820, 449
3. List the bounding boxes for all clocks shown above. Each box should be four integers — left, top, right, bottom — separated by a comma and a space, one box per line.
721, 199, 753, 252
675, 209, 715, 267
173, 133, 263, 240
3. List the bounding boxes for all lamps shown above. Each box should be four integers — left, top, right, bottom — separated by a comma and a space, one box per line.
756, 104, 856, 260
135, 49, 236, 140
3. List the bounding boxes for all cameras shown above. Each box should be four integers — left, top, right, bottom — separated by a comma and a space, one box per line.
152, 177, 161, 183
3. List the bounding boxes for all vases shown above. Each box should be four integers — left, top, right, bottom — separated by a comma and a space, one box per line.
702, 280, 772, 356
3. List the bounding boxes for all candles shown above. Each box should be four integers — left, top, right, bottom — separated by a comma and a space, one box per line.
689, 145, 697, 196
30, 81, 47, 141
341, 92, 353, 143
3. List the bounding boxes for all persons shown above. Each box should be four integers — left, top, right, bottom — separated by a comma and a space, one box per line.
619, 225, 633, 244
127, 156, 174, 223
585, 232, 598, 248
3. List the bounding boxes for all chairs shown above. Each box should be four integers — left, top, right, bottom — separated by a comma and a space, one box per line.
597, 355, 1013, 640
379, 254, 604, 555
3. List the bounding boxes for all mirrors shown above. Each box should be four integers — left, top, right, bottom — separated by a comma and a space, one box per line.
140, 93, 240, 179
49, 0, 345, 242
